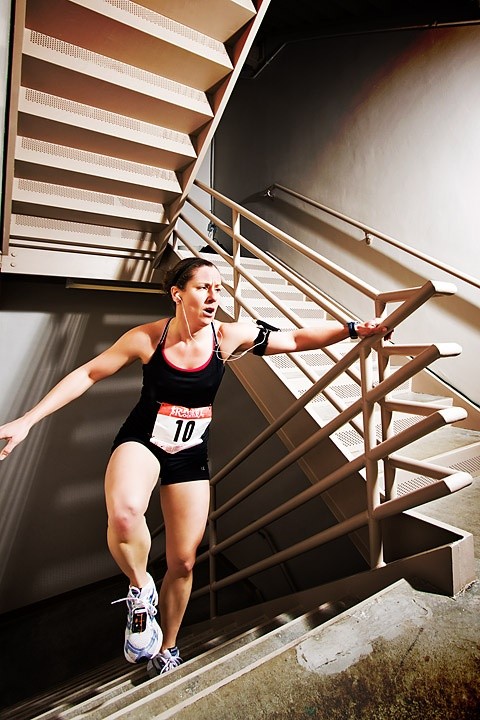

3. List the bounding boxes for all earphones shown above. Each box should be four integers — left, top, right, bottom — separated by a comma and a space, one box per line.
174, 292, 181, 300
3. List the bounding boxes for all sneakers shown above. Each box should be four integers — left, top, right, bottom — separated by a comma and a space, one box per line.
147, 646, 183, 679
111, 571, 163, 663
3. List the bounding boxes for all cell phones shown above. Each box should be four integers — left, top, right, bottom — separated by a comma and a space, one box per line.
256, 320, 281, 332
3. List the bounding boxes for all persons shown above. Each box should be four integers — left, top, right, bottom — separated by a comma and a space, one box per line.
0, 259, 395, 678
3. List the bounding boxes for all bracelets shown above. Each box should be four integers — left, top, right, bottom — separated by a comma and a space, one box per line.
346, 322, 360, 339
